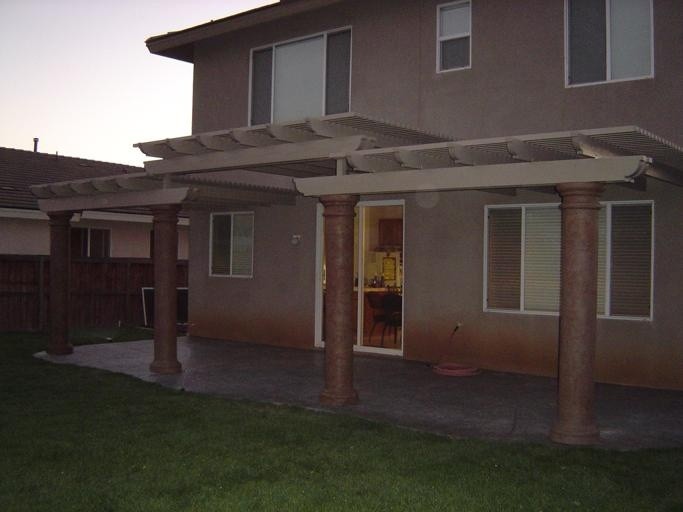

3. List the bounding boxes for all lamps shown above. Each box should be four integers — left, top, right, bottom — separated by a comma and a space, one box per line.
290, 234, 300, 246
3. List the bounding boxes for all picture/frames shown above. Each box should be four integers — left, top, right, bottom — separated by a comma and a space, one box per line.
383, 257, 396, 280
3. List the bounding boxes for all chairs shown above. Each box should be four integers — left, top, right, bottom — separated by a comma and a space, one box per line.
367, 292, 402, 345
